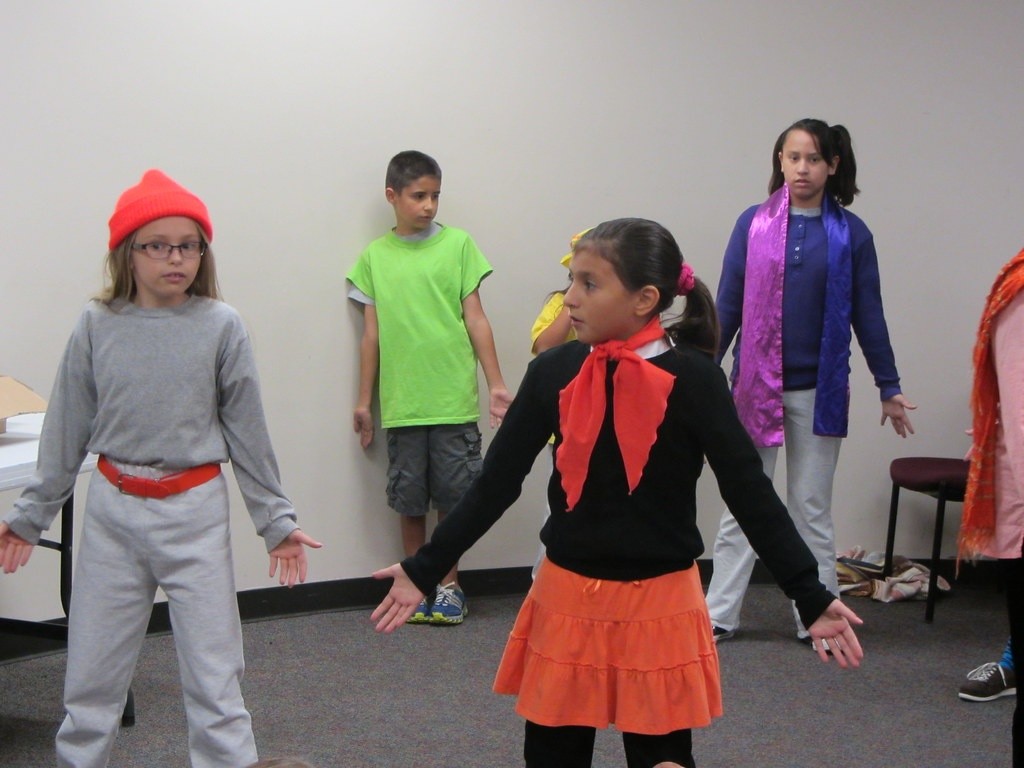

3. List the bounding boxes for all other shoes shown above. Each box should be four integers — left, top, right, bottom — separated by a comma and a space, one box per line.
712, 626, 736, 642
801, 632, 844, 650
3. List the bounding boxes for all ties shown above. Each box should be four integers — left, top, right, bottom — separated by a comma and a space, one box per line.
557, 315, 676, 511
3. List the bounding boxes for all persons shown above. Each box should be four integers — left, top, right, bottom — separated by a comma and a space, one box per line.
957, 247, 1024, 768
346, 150, 513, 622
705, 119, 915, 650
371, 218, 863, 768
0, 168, 321, 767
530, 228, 592, 443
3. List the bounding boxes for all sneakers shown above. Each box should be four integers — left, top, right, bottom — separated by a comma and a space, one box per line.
958, 659, 1018, 700
405, 597, 430, 623
428, 579, 469, 624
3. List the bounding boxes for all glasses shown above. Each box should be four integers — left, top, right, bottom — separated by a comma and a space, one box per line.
131, 241, 207, 260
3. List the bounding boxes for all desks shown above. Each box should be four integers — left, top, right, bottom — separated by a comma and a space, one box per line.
1, 433, 136, 726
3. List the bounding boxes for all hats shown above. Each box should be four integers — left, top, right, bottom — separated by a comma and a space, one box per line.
561, 226, 596, 268
108, 170, 212, 253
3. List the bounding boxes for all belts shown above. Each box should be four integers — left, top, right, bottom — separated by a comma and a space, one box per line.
98, 454, 222, 498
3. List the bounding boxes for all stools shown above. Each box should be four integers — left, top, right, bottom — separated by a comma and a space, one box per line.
881, 454, 973, 623
